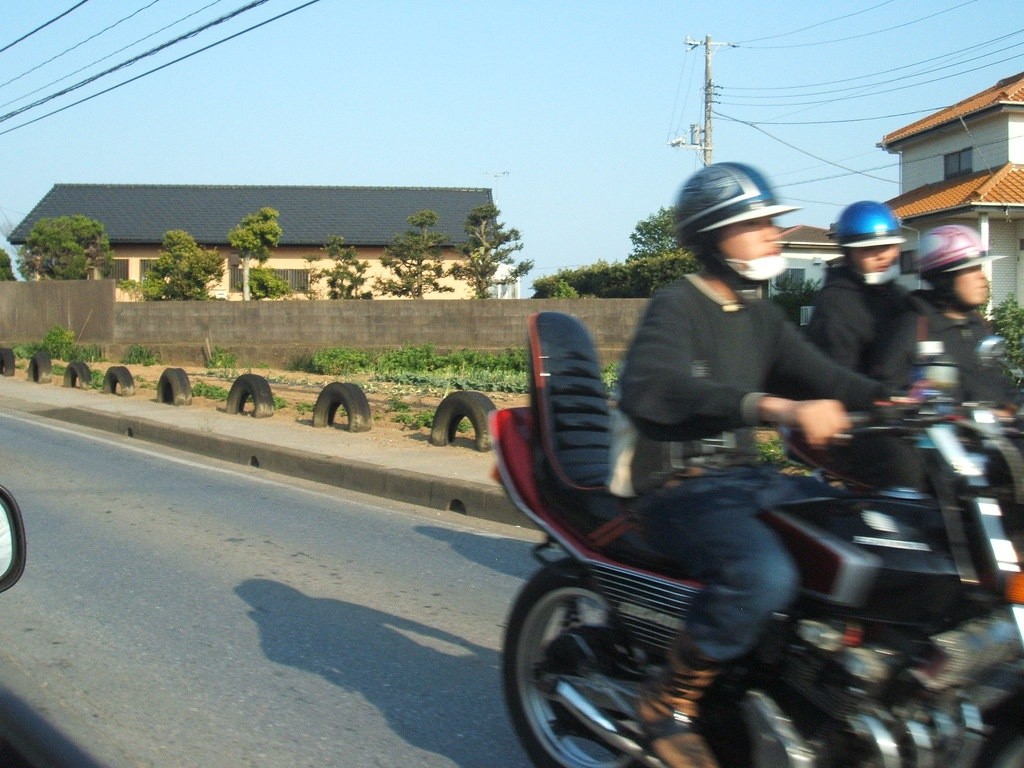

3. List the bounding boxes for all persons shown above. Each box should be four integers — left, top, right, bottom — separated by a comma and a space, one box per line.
782, 199, 1023, 505
617, 162, 1011, 768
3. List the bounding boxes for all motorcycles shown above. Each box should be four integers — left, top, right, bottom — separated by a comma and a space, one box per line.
487, 310, 1023, 768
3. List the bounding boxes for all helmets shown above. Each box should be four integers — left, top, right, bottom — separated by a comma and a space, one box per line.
912, 225, 1010, 272
672, 162, 803, 245
837, 201, 908, 247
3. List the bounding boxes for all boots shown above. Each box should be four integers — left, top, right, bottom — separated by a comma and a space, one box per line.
634, 649, 726, 768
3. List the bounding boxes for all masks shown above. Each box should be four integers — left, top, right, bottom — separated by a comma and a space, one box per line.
852, 263, 903, 285
724, 254, 789, 281
